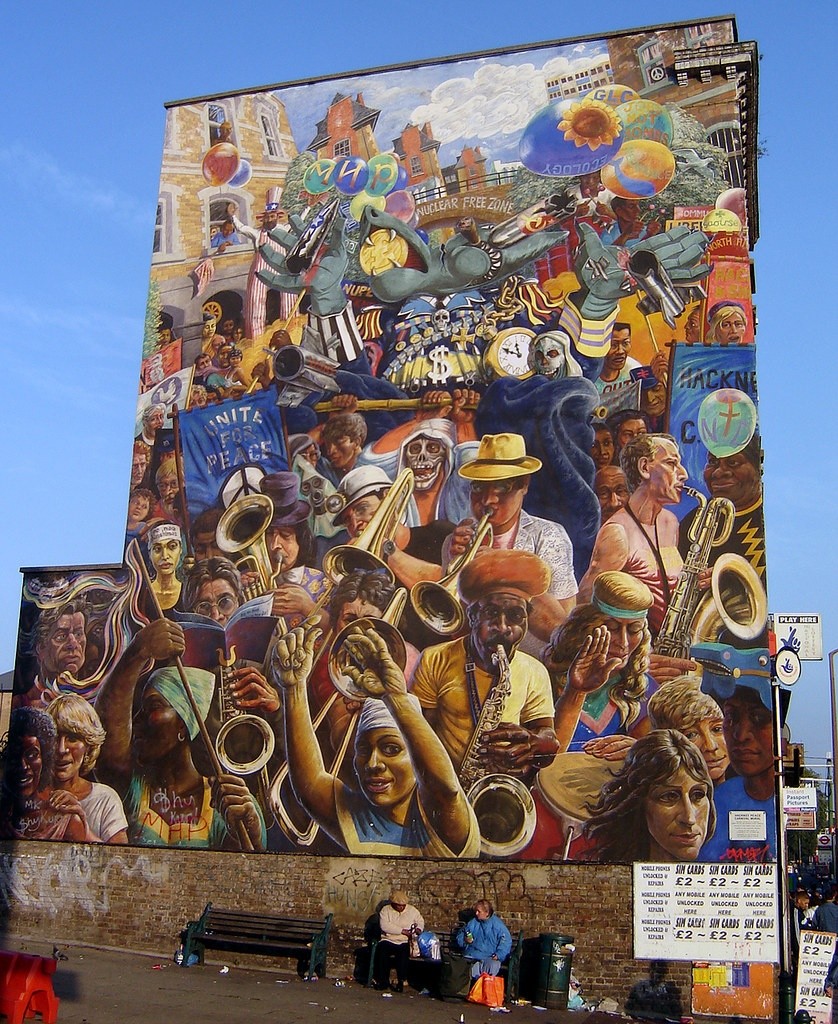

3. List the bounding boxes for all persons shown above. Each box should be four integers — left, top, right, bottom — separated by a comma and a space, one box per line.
456, 899, 512, 980
789, 882, 838, 998
372, 892, 424, 993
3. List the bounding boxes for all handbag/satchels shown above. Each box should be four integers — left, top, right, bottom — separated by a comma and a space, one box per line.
467, 972, 505, 1006
410, 924, 442, 961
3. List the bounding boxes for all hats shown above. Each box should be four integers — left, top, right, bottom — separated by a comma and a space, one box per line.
389, 891, 409, 905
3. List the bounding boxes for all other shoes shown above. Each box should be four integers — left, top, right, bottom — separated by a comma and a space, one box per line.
373, 982, 389, 989
396, 982, 403, 992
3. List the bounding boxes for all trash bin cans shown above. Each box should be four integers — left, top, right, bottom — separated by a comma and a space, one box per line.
532, 933, 574, 1009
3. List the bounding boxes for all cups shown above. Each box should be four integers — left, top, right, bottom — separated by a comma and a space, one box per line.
467, 933, 473, 942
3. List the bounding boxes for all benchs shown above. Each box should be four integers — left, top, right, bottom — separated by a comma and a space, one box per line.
369, 930, 526, 1004
181, 902, 334, 980
